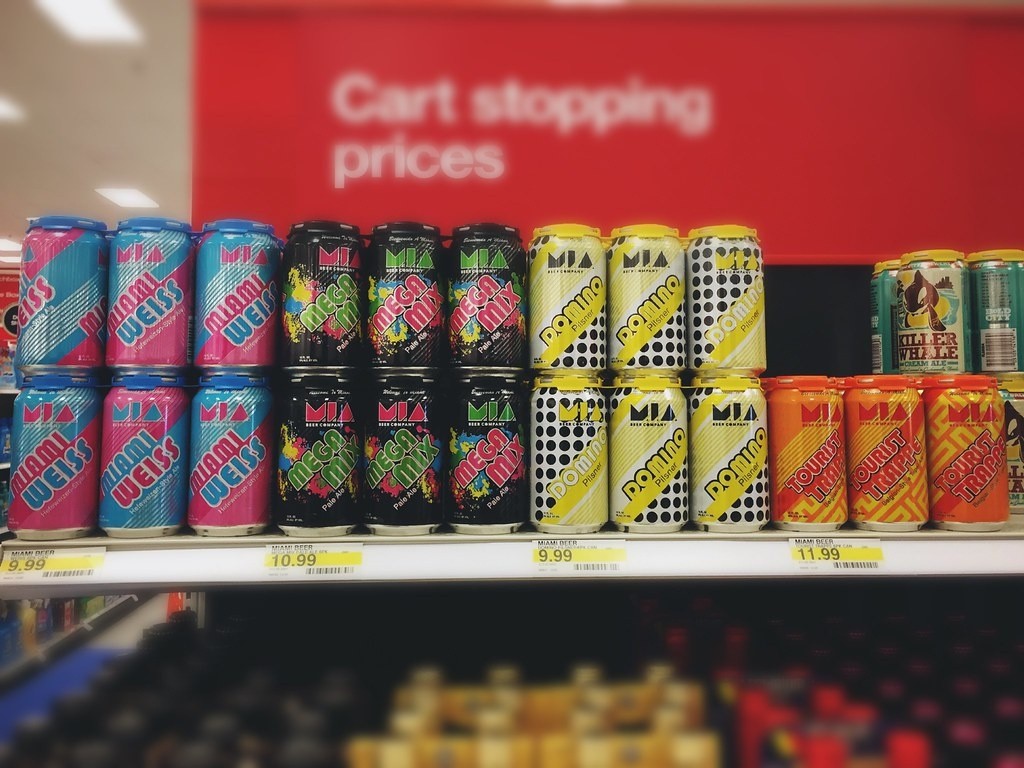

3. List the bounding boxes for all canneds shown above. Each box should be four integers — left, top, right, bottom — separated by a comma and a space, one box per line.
3, 209, 1024, 549
